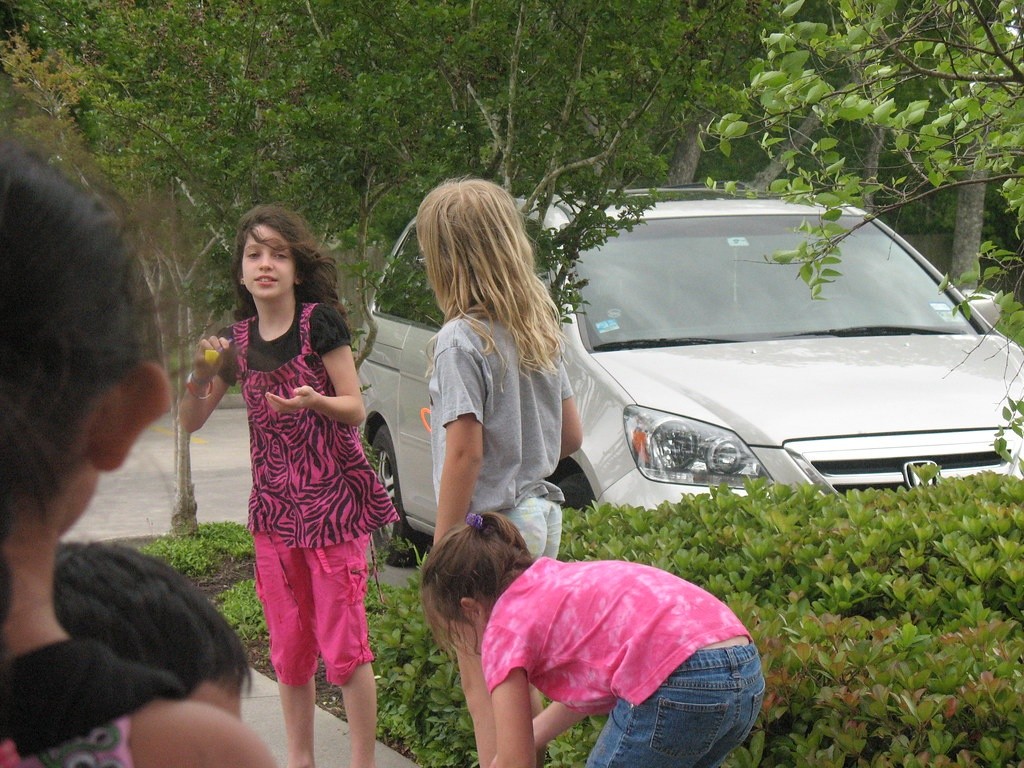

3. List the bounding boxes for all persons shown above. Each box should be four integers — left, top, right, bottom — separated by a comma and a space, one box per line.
420, 510, 766, 768
414, 176, 584, 768
178, 205, 400, 768
0, 132, 279, 768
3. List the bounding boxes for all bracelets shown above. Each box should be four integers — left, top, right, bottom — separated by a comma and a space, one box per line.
187, 371, 214, 400
192, 373, 213, 385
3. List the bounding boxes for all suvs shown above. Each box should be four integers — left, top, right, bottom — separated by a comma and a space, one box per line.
345, 185, 1024, 568
